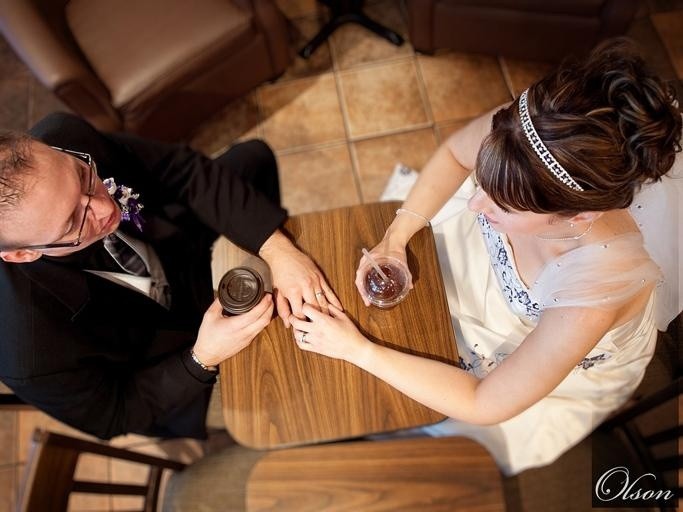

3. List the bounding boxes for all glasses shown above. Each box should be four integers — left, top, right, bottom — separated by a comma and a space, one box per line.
16, 145, 98, 254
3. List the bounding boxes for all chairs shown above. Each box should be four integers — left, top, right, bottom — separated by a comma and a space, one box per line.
505, 381, 682, 512
13, 428, 266, 512
409, 1, 639, 67
0, 0, 297, 146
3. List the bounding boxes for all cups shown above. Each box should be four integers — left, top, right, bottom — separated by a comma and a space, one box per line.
215, 266, 264, 315
362, 257, 410, 313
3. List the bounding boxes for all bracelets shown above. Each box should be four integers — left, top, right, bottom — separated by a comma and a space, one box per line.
188, 348, 218, 375
395, 207, 430, 228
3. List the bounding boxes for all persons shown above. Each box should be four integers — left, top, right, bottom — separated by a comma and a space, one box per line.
0, 111, 344, 441
286, 33, 683, 478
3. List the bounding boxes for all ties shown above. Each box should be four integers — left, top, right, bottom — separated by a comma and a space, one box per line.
102, 232, 150, 280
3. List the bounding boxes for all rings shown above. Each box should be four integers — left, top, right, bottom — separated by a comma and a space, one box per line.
315, 290, 324, 295
302, 332, 307, 343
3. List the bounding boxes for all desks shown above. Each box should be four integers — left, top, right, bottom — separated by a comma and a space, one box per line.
220, 201, 462, 450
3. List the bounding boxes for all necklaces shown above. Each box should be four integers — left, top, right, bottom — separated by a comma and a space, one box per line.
534, 221, 596, 241
